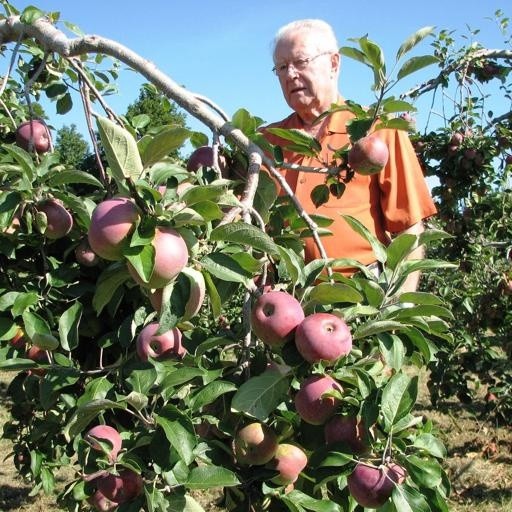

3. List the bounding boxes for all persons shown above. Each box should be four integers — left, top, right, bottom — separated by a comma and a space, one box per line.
229, 19, 439, 454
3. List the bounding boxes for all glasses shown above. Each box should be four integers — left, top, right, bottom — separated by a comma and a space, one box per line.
272, 52, 331, 76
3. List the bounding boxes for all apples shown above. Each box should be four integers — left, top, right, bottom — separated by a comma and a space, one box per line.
9, 112, 512, 512
